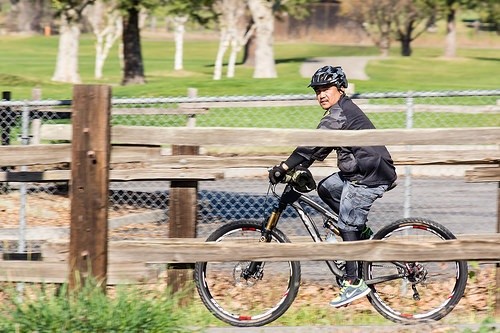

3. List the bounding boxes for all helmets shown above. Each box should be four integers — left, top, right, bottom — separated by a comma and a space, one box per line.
308, 66, 348, 90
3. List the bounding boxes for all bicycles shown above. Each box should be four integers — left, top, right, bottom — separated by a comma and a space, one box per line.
194, 161, 469, 328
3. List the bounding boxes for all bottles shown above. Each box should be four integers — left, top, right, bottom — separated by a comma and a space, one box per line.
326, 235, 345, 263
323, 219, 340, 234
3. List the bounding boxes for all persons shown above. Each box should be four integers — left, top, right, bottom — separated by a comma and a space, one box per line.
268, 65, 397, 308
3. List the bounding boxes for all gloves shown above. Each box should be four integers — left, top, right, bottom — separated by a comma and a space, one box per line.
269, 166, 285, 185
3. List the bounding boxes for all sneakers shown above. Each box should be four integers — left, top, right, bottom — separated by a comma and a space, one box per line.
329, 278, 371, 307
